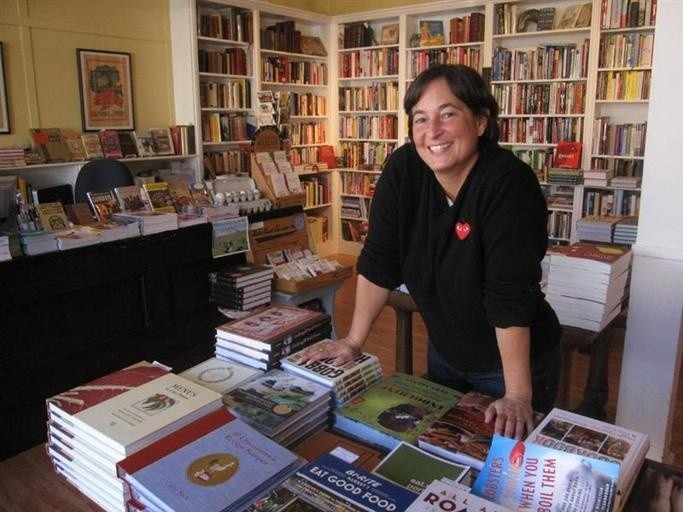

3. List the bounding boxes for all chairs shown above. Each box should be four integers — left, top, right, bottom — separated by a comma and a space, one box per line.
75, 159, 134, 214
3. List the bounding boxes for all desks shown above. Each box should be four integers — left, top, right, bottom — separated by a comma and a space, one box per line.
0, 428, 683, 512
272, 281, 345, 342
384, 289, 620, 420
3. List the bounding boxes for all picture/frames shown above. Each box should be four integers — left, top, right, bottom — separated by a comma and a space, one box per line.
0, 41, 10, 134
76, 47, 135, 133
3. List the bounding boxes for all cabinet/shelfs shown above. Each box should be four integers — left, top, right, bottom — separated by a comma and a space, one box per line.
336, 0, 657, 246
170, 0, 336, 257
